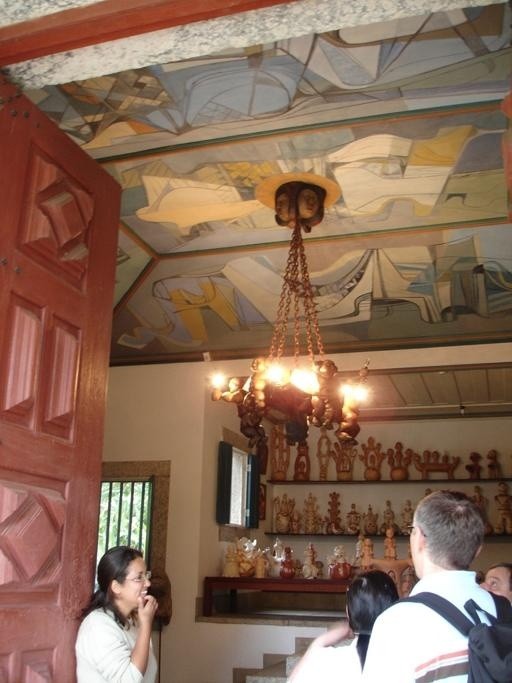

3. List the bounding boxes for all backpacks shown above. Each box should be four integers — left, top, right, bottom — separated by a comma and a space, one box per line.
402, 591, 512, 682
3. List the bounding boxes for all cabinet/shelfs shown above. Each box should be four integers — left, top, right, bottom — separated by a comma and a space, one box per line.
201, 576, 353, 628
262, 476, 512, 544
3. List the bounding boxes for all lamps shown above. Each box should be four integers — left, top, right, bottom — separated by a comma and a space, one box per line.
204, 178, 373, 450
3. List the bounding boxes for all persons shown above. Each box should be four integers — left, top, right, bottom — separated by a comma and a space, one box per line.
479, 561, 511, 604
284, 569, 400, 683
74, 545, 159, 682
358, 489, 511, 682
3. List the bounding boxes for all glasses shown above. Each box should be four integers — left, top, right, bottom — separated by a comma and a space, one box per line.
120, 570, 152, 582
407, 524, 425, 537
